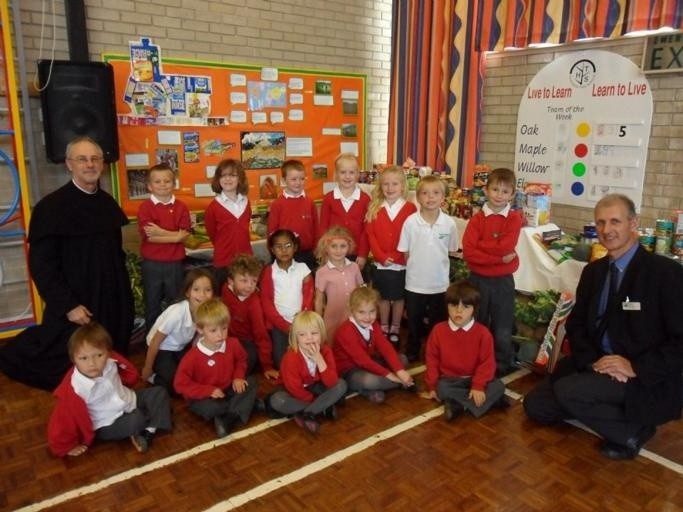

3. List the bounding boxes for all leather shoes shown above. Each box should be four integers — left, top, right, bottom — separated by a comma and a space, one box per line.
601, 424, 657, 460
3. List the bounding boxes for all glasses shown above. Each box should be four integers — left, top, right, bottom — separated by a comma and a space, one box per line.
68, 154, 104, 164
219, 172, 239, 179
272, 242, 295, 252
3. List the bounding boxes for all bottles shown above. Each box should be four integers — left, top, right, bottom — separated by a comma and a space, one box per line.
576, 232, 592, 262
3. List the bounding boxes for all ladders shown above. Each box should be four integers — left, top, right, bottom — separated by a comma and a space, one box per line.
0, 0, 43, 340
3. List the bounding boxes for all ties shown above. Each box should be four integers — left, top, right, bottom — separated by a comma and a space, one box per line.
598, 261, 619, 338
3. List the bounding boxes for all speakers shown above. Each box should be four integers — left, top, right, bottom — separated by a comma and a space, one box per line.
38, 60, 119, 164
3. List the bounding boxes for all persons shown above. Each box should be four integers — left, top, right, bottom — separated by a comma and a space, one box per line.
524, 194, 682, 462
24, 137, 522, 459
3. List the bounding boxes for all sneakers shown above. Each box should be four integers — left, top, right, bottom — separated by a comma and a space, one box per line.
293, 413, 319, 432
253, 398, 265, 411
495, 360, 512, 376
323, 403, 338, 420
358, 389, 386, 404
128, 429, 153, 454
444, 398, 463, 421
494, 397, 511, 410
406, 341, 420, 361
214, 414, 228, 438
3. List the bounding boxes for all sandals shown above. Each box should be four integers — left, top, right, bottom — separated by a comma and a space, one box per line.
389, 330, 401, 351
